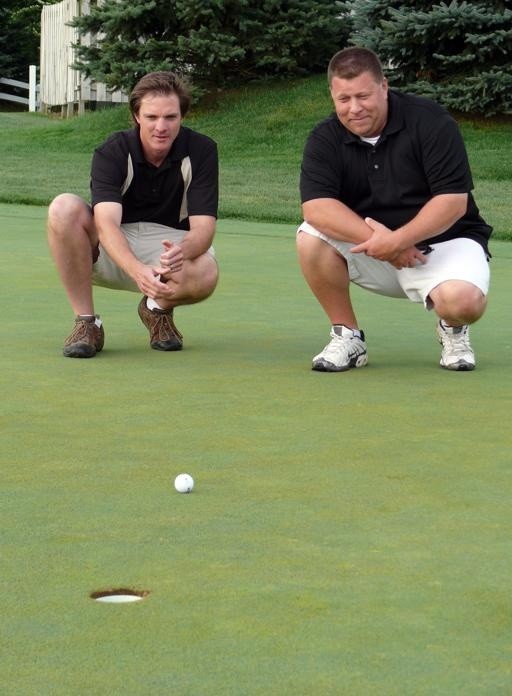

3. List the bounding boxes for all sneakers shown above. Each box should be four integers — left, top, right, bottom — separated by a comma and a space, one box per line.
138, 294, 182, 351
436, 320, 475, 370
312, 325, 367, 371
63, 315, 104, 358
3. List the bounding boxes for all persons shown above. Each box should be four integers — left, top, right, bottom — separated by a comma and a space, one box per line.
46, 71, 218, 359
296, 39, 491, 371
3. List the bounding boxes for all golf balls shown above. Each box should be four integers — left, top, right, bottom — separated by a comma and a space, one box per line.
175, 474, 193, 493
